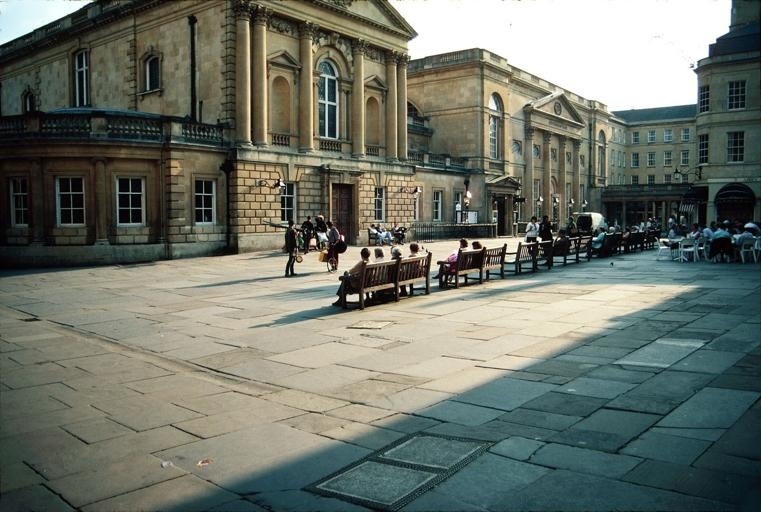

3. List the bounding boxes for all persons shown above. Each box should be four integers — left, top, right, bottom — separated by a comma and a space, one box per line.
524, 215, 539, 255
538, 214, 553, 256
567, 215, 578, 247
332, 248, 370, 305
315, 215, 325, 249
586, 214, 760, 263
301, 216, 312, 254
367, 221, 405, 246
400, 242, 420, 297
387, 246, 403, 297
466, 241, 483, 270
431, 239, 468, 280
284, 220, 299, 277
542, 229, 570, 266
366, 247, 386, 302
325, 220, 340, 271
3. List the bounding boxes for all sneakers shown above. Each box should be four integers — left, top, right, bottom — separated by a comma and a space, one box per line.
432, 274, 442, 279
376, 241, 404, 246
285, 274, 292, 278
290, 273, 297, 276
364, 290, 408, 303
332, 299, 346, 306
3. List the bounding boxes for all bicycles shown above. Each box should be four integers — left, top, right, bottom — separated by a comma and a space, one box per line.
326, 244, 339, 274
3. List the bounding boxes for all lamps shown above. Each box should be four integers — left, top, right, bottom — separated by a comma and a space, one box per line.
581, 200, 588, 207
400, 186, 422, 195
260, 179, 286, 189
553, 197, 559, 207
568, 197, 574, 207
535, 196, 543, 206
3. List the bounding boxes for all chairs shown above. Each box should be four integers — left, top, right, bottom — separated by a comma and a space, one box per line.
655, 236, 761, 263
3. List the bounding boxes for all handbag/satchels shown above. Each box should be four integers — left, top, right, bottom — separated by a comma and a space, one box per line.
309, 235, 317, 247
333, 231, 347, 254
319, 246, 330, 262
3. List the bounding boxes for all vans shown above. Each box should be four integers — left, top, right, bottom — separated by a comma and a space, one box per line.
572, 212, 610, 238
